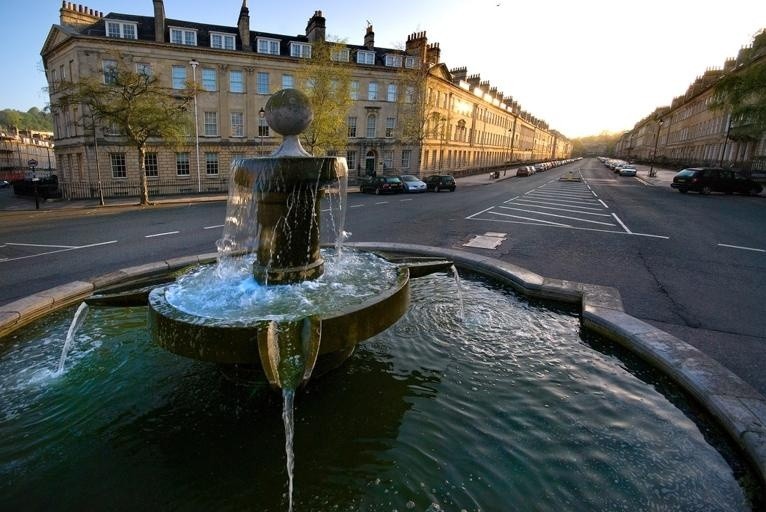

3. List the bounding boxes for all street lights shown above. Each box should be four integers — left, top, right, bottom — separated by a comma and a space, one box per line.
502, 128, 511, 176
624, 129, 635, 165
648, 119, 666, 177
85, 98, 104, 206
257, 107, 265, 157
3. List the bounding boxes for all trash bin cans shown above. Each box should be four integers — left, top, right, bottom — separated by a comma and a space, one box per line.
495, 171, 499, 179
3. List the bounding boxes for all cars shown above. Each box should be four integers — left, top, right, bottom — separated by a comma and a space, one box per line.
672, 166, 764, 198
359, 173, 458, 194
595, 154, 639, 177
0, 180, 9, 188
516, 154, 583, 181
629, 158, 637, 165
